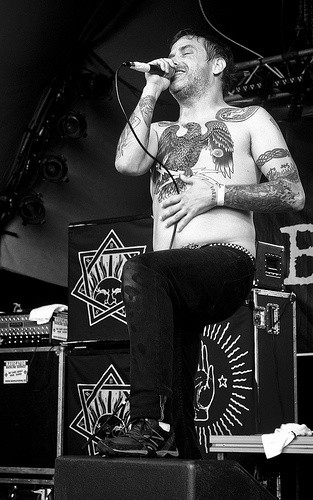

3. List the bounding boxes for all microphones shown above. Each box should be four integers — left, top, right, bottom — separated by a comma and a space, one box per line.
127, 61, 175, 79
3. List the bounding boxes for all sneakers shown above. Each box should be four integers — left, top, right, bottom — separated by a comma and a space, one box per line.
98, 417, 179, 458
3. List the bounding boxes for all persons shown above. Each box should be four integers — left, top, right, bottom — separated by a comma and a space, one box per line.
96, 28, 306, 458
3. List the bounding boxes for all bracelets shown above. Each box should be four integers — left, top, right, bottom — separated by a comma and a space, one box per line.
216, 181, 224, 207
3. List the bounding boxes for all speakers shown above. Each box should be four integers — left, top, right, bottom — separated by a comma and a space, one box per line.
53, 455, 279, 500
